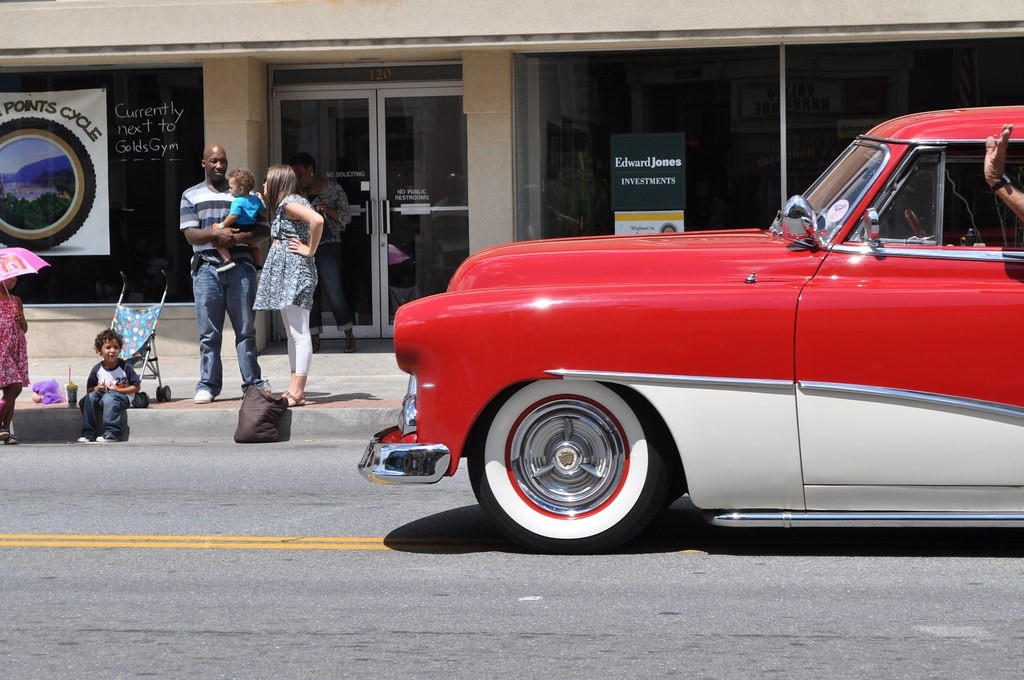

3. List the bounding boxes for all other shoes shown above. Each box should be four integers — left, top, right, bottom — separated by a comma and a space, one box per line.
96, 435, 118, 442
194, 390, 214, 403
312, 338, 322, 354
0, 425, 10, 440
216, 258, 236, 272
343, 334, 355, 353
4, 431, 17, 444
76, 435, 95, 442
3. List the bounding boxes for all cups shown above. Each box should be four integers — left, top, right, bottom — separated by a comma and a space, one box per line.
66, 384, 78, 408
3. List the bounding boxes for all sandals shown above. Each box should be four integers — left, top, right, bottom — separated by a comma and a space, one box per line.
280, 391, 306, 407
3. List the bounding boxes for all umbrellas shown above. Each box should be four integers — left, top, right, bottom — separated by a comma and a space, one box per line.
0, 247, 51, 317
388, 244, 410, 266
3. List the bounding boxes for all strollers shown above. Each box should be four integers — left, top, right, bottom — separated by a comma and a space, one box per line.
109, 270, 171, 408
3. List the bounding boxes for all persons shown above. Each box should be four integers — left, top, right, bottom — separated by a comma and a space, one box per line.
210, 168, 268, 274
180, 145, 270, 405
289, 153, 357, 354
252, 165, 324, 408
984, 121, 1020, 217
77, 328, 141, 443
0, 276, 30, 446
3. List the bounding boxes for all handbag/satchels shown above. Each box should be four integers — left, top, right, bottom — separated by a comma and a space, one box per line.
234, 380, 289, 443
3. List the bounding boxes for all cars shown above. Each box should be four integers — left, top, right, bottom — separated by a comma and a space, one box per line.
359, 103, 1024, 555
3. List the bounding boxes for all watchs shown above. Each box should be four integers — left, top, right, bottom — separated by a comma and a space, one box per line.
990, 172, 1011, 192
